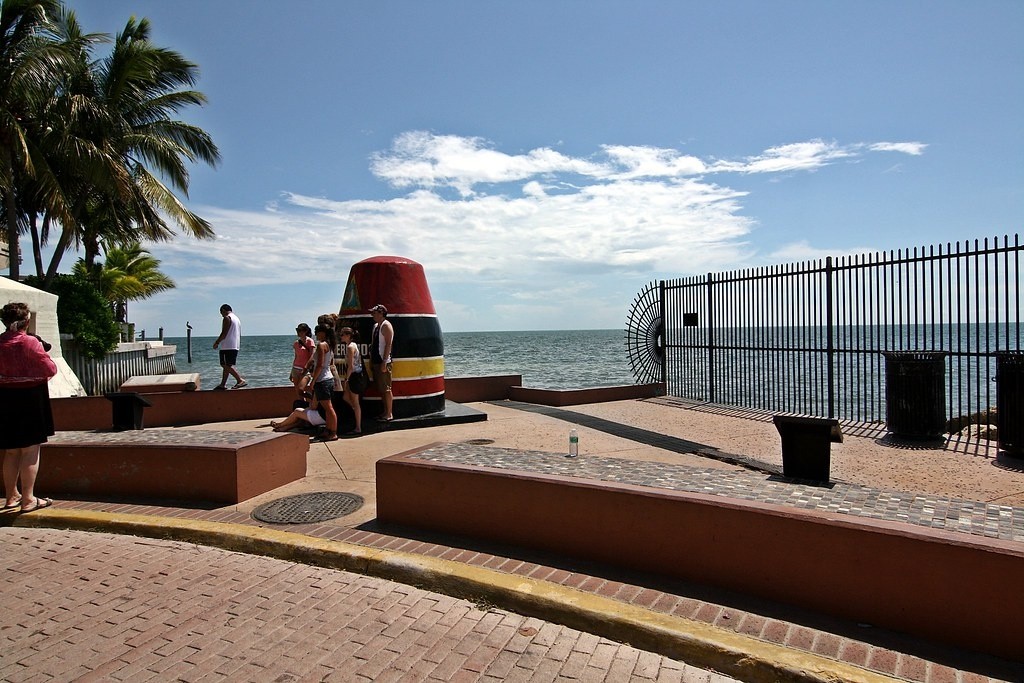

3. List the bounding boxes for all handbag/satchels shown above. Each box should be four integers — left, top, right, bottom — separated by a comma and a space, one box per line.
309, 390, 318, 410
349, 373, 370, 395
309, 364, 314, 373
330, 365, 343, 392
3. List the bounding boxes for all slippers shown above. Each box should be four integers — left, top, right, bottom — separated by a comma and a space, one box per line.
232, 384, 247, 389
377, 417, 393, 421
374, 416, 382, 420
213, 386, 227, 389
346, 431, 361, 435
20, 498, 52, 513
5, 501, 22, 508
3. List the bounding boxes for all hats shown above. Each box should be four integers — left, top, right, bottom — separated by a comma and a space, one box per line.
368, 305, 387, 315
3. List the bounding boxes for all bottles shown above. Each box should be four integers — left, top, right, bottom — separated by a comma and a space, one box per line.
568, 428, 578, 457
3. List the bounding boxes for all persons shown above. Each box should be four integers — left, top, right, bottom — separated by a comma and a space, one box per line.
0, 302, 58, 512
367, 304, 394, 422
270, 313, 344, 442
212, 304, 247, 392
340, 327, 364, 436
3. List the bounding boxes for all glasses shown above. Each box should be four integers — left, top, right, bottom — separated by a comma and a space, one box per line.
296, 328, 300, 331
340, 333, 343, 337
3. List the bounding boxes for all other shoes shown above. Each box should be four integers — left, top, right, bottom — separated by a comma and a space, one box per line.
273, 428, 282, 431
271, 421, 280, 428
